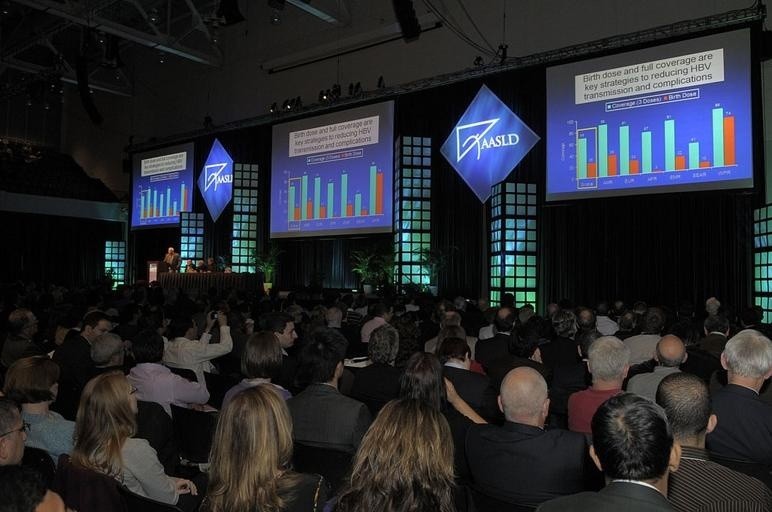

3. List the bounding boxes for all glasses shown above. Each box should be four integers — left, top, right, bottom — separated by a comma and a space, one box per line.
0, 422, 31, 438
130, 387, 139, 396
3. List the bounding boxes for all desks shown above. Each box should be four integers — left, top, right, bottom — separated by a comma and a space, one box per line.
157, 272, 265, 299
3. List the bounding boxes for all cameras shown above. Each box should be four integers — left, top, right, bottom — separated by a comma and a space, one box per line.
212, 313, 218, 319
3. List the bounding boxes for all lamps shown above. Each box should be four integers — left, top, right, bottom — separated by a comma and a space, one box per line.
269, 74, 385, 119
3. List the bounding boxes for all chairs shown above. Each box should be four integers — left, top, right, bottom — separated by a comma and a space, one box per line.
1, 300, 772, 512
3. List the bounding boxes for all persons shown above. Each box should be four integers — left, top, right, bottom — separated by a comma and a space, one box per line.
161, 246, 230, 274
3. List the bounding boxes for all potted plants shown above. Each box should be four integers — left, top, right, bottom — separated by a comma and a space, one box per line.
347, 251, 375, 293
411, 247, 449, 296
249, 246, 287, 294
